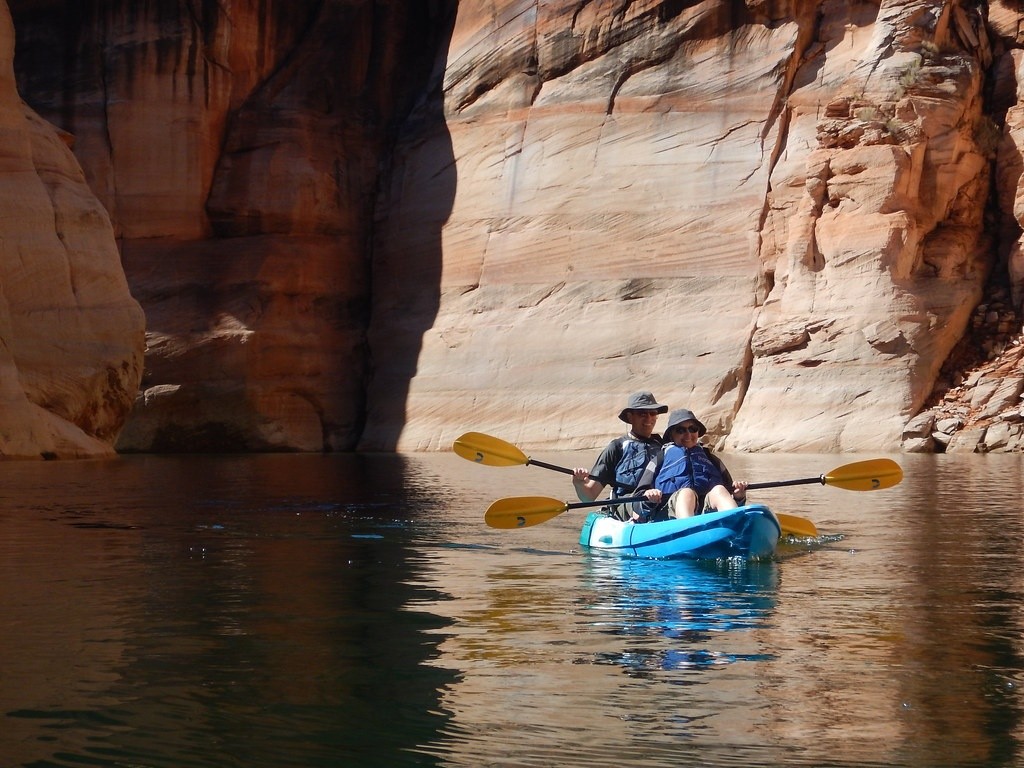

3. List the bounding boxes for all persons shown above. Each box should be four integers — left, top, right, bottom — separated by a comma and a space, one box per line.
573, 391, 668, 524
632, 409, 748, 519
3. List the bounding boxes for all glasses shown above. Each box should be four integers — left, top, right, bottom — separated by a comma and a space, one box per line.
631, 411, 659, 418
676, 424, 700, 434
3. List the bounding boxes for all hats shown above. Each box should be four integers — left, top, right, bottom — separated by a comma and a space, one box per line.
619, 392, 668, 424
663, 409, 707, 442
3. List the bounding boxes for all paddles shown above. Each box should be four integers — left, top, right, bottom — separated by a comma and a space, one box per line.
483, 457, 905, 531
451, 431, 818, 544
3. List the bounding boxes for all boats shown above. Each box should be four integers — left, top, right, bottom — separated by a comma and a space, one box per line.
579, 503, 785, 563
